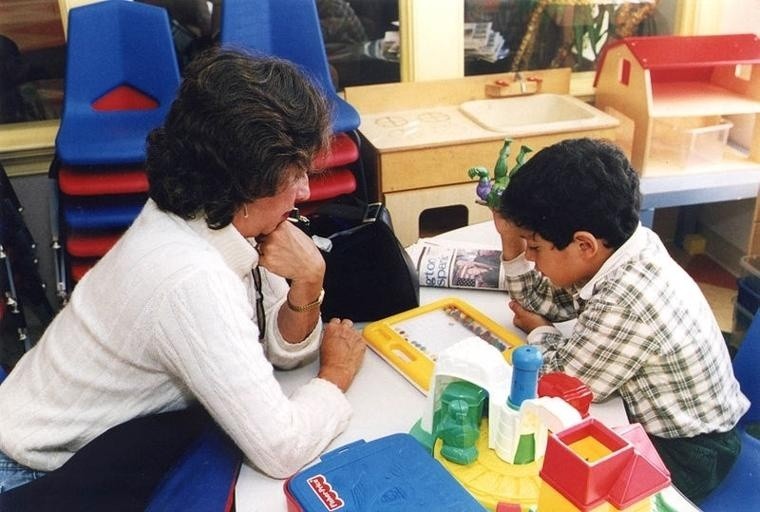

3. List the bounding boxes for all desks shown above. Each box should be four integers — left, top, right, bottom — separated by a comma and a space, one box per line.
235, 286, 630, 512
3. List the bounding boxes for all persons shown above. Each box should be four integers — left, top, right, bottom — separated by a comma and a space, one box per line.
466, 138, 531, 215
0, 45, 365, 510
453, 249, 502, 289
313, 1, 368, 94
489, 137, 751, 500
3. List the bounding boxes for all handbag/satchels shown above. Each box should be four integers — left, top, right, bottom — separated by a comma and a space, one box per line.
291, 205, 422, 327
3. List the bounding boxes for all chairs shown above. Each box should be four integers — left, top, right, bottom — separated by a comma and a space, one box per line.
695, 308, 760, 512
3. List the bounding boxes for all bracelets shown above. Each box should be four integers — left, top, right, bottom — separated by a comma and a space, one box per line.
286, 286, 326, 312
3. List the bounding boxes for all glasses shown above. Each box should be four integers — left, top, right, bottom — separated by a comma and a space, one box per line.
251, 264, 266, 342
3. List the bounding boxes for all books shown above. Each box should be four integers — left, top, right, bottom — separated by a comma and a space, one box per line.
463, 20, 507, 64
